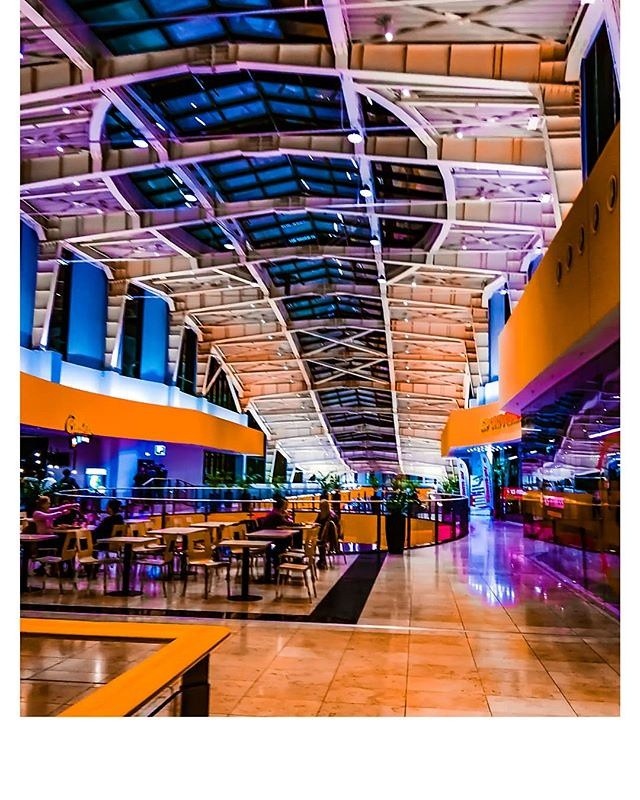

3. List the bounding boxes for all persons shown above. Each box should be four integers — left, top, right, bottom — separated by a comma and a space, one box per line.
31, 462, 167, 580
270, 499, 336, 570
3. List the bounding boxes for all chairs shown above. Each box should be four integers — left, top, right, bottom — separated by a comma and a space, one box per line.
33, 520, 347, 604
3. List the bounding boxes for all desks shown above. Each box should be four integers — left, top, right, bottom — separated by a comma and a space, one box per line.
218, 540, 272, 602
97, 537, 158, 597
20, 535, 58, 595
276, 525, 316, 564
146, 527, 208, 580
55, 526, 97, 533
190, 522, 239, 541
246, 529, 299, 585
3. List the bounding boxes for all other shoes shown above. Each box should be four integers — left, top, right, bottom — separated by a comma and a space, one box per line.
316, 560, 327, 569
